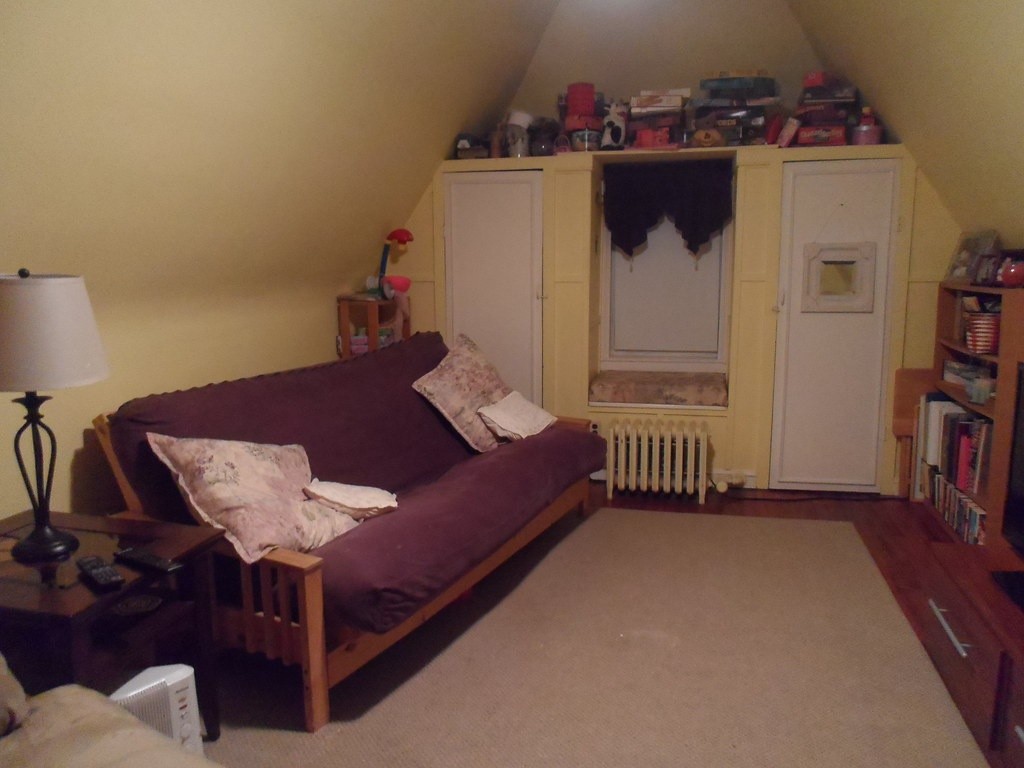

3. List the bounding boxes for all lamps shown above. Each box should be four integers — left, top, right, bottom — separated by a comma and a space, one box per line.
348, 229, 414, 300
0, 269, 107, 562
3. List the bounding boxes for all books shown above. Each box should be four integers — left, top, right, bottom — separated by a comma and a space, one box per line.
915, 392, 992, 544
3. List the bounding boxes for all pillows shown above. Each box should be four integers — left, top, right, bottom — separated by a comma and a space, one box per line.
476, 391, 559, 440
413, 334, 514, 453
146, 432, 364, 564
304, 477, 399, 519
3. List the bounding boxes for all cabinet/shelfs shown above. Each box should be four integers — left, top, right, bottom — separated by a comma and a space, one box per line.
337, 292, 410, 358
917, 279, 1024, 768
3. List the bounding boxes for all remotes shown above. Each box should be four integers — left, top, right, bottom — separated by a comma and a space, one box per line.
76, 554, 124, 589
113, 546, 184, 572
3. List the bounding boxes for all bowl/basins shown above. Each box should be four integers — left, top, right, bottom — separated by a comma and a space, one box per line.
852, 126, 883, 144
571, 129, 602, 151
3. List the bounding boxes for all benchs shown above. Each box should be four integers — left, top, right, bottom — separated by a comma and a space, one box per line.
91, 330, 609, 731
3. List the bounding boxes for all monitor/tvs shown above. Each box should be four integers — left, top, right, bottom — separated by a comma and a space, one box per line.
991, 361, 1024, 608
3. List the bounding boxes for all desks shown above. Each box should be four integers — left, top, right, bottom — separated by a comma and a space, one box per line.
0, 508, 227, 740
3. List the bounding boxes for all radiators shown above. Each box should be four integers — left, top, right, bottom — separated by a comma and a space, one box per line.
607, 418, 709, 505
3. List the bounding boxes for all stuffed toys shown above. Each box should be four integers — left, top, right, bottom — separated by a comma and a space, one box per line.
600, 97, 630, 150
